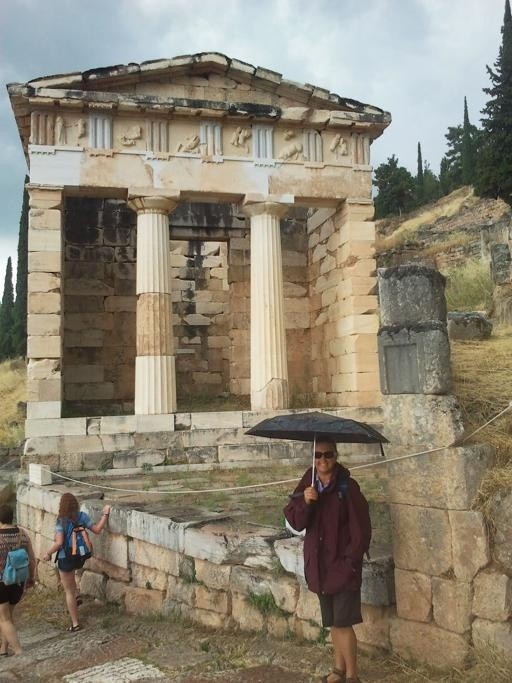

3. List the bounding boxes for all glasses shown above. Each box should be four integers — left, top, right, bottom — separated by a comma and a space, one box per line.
315, 451, 334, 458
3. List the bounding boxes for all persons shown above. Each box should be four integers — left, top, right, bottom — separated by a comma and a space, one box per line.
43, 493, 110, 632
283, 436, 372, 683
0, 505, 36, 658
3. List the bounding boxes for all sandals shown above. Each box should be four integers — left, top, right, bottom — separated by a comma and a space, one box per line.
66, 623, 80, 630
322, 667, 346, 682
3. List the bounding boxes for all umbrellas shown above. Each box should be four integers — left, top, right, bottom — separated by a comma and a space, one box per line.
243, 411, 390, 506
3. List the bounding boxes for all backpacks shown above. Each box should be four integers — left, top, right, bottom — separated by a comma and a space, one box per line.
63, 520, 93, 559
0, 527, 29, 585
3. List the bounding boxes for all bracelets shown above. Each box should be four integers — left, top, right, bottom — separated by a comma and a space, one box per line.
102, 512, 110, 519
